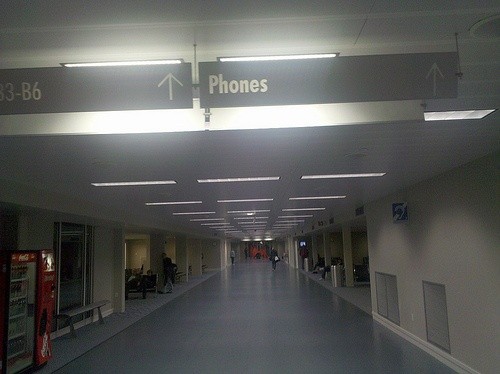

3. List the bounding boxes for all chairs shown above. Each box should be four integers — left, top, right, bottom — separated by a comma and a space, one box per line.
355, 265, 369, 288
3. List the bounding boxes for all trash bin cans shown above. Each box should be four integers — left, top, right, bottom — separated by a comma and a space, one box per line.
304, 258, 312, 272
331, 265, 343, 286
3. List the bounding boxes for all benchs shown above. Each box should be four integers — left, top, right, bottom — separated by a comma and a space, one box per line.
56, 300, 110, 338
128, 272, 158, 297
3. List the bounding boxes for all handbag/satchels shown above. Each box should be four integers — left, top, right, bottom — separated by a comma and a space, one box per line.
275, 257, 280, 263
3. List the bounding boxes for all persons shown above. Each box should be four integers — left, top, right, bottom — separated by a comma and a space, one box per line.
230, 247, 236, 265
318, 265, 332, 280
298, 244, 309, 270
162, 253, 176, 293
313, 254, 325, 270
269, 247, 280, 273
337, 258, 343, 265
125, 264, 156, 293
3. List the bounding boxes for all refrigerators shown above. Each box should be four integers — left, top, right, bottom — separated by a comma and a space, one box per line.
0, 248, 54, 374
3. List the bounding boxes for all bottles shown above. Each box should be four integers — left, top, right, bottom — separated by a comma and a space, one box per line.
7, 338, 24, 362
9, 282, 23, 297
8, 316, 25, 336
10, 265, 27, 279
9, 298, 25, 316
